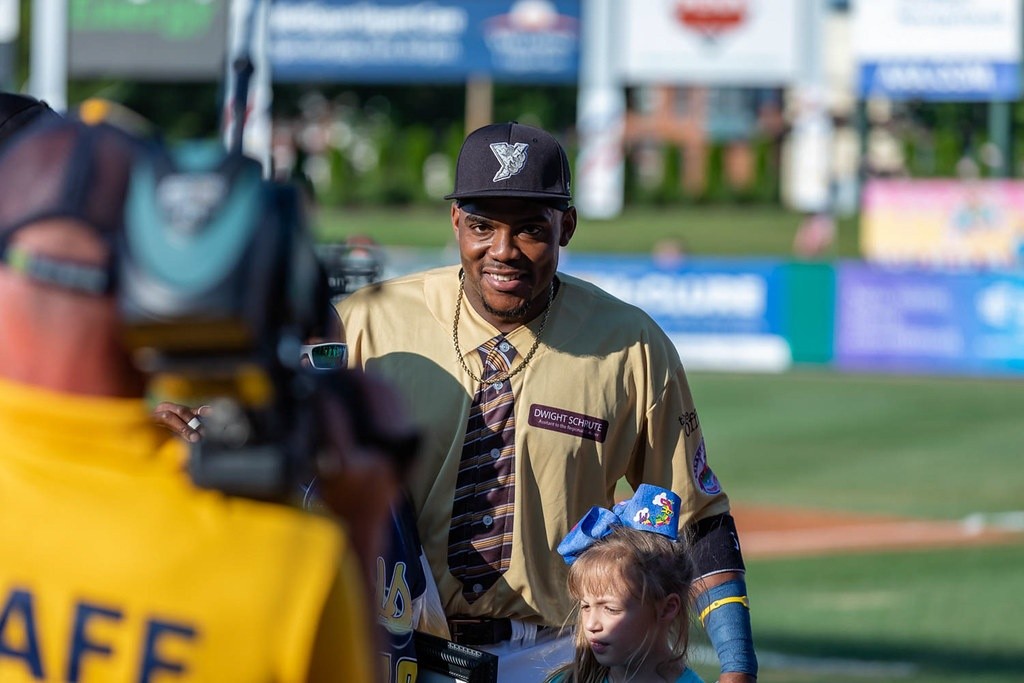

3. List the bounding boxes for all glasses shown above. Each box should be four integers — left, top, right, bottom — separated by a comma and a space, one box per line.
299, 342, 348, 370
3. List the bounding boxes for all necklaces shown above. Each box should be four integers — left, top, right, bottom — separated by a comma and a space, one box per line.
452, 272, 554, 385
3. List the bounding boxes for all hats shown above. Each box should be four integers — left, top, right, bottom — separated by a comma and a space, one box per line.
52, 98, 161, 156
444, 122, 572, 200
0, 92, 61, 143
0, 112, 145, 291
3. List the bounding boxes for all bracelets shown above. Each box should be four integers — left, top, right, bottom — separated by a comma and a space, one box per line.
698, 595, 750, 627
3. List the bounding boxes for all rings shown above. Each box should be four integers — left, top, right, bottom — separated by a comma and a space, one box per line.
196, 404, 211, 415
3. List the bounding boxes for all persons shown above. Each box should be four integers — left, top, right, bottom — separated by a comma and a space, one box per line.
333, 120, 758, 683
542, 483, 708, 683
0, 91, 453, 683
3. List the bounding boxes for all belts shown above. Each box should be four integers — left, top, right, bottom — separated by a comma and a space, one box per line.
447, 616, 550, 645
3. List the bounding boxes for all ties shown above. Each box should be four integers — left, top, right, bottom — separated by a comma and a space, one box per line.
445, 334, 516, 607
